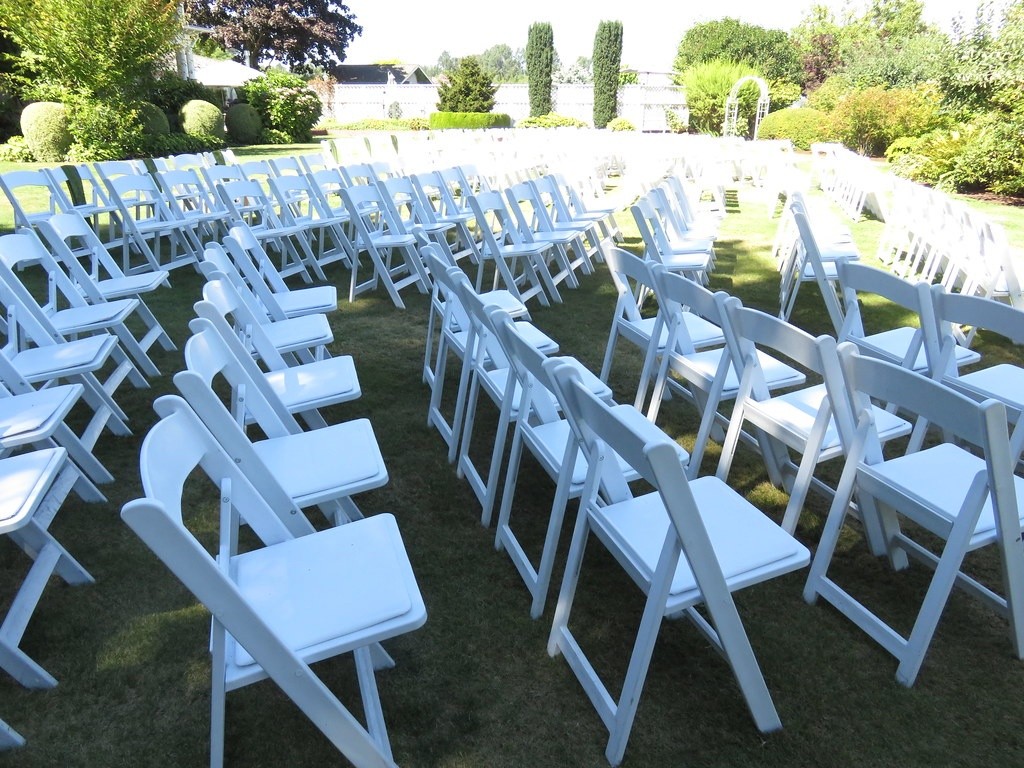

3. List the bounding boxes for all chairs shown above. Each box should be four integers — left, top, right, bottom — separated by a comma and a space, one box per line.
0, 128, 1024, 768
